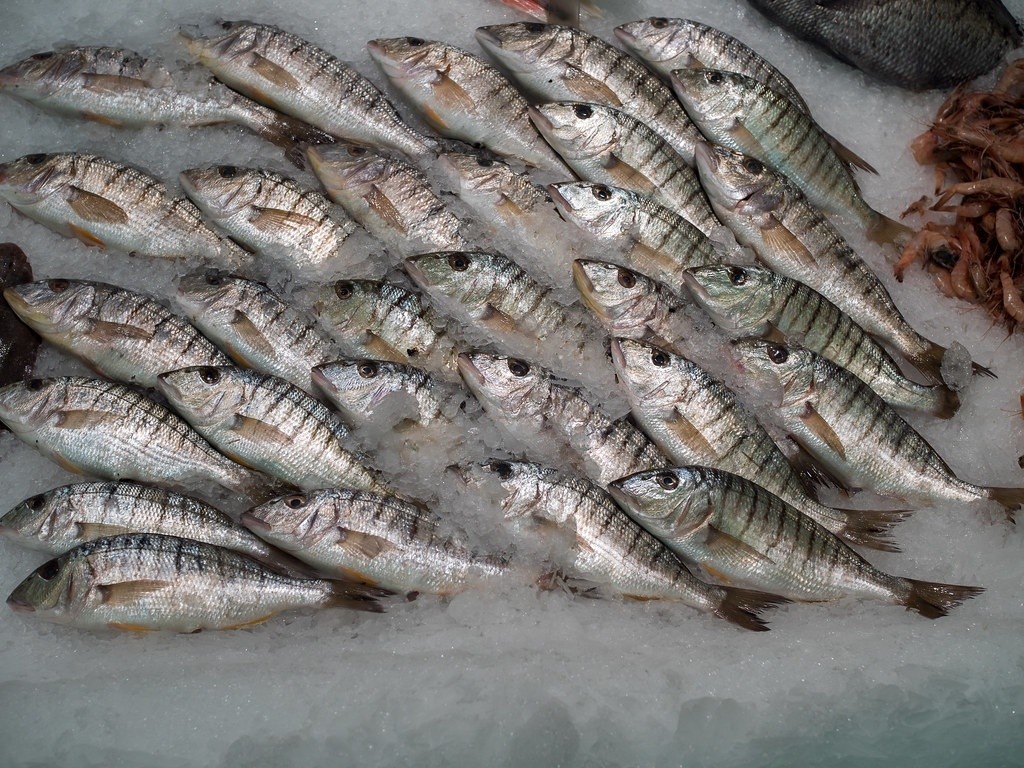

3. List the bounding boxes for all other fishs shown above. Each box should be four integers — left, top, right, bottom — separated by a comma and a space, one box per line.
0, 0, 1024, 635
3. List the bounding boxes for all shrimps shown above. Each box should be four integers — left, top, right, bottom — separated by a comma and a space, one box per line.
887, 44, 1024, 474
